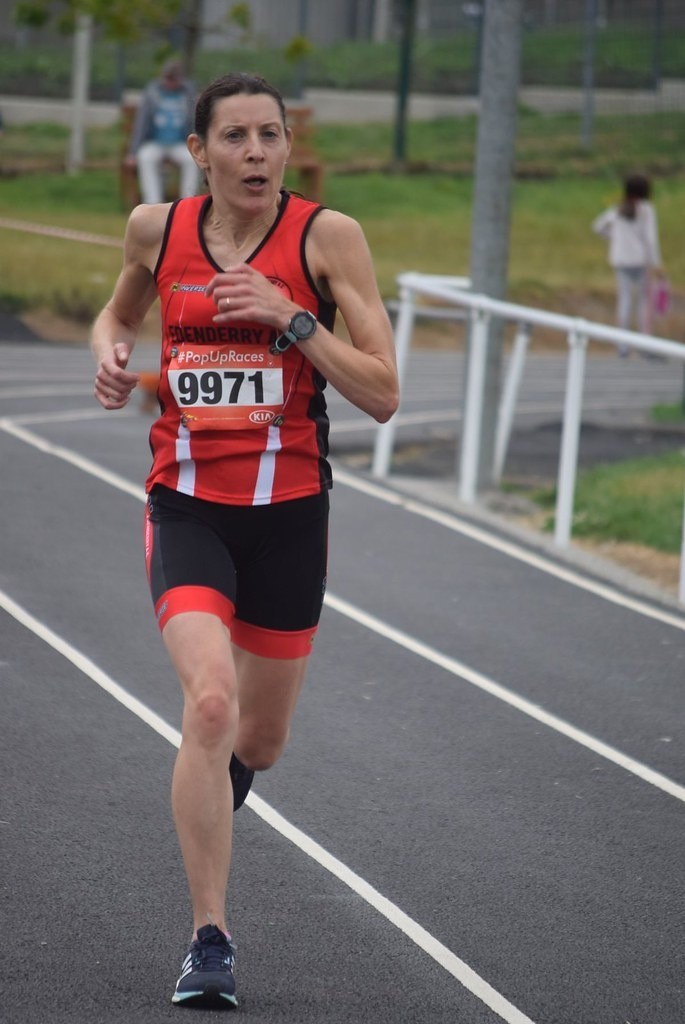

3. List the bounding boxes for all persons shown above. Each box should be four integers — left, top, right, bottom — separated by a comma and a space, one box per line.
125, 60, 199, 205
591, 176, 664, 358
90, 72, 401, 1010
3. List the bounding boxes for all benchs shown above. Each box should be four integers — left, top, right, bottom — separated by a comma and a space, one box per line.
120, 93, 323, 216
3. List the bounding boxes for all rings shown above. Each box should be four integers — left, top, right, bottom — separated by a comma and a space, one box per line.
226, 297, 230, 306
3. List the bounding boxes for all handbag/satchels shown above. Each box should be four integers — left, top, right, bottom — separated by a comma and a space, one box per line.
653, 285, 668, 313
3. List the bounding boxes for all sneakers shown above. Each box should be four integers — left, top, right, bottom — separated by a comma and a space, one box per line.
229, 751, 254, 813
172, 925, 238, 1009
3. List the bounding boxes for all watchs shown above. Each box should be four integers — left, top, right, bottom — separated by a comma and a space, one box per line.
277, 309, 317, 353
